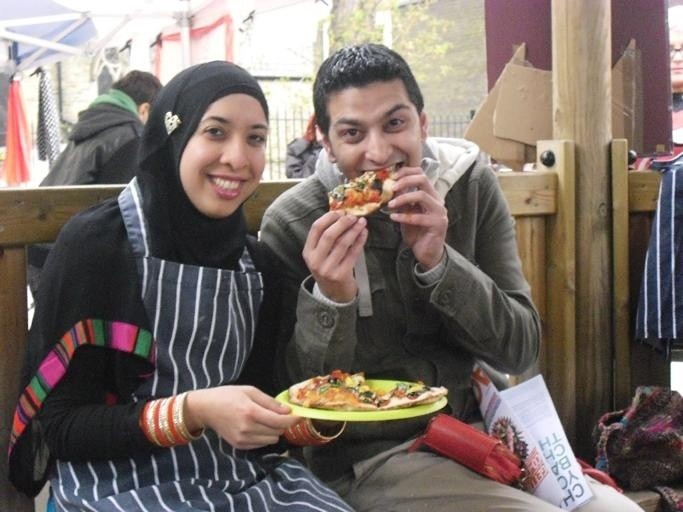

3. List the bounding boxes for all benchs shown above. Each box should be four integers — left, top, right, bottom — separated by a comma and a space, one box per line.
609, 135, 681, 496
0, 138, 657, 510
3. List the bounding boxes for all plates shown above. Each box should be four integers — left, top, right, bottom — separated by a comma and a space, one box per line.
272, 380, 449, 422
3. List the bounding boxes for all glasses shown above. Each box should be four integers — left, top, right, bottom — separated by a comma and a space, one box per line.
669, 43, 683, 58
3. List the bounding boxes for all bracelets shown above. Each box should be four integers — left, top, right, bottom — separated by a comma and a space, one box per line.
143, 391, 205, 448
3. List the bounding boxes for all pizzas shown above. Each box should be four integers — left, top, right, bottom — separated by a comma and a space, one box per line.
326, 166, 396, 217
288, 370, 448, 411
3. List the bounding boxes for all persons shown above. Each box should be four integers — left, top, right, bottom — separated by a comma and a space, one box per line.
667, 6, 683, 145
10, 61, 358, 512
27, 70, 164, 303
261, 42, 641, 512
286, 111, 323, 178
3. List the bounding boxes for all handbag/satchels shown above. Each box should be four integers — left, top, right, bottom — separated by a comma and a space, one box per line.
406, 413, 526, 487
